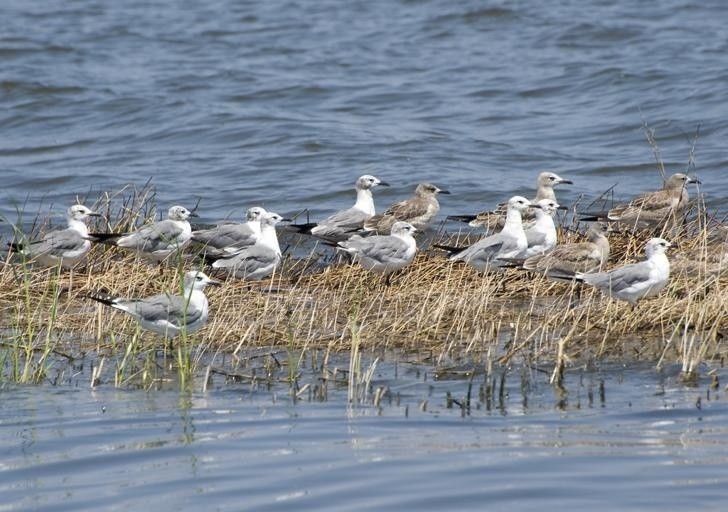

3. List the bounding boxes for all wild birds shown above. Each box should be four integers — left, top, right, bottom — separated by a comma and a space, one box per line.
537, 237, 682, 317
576, 173, 702, 242
290, 170, 574, 294
85, 270, 222, 350
496, 220, 623, 300
6, 204, 102, 273
190, 206, 293, 294
81, 205, 199, 276
